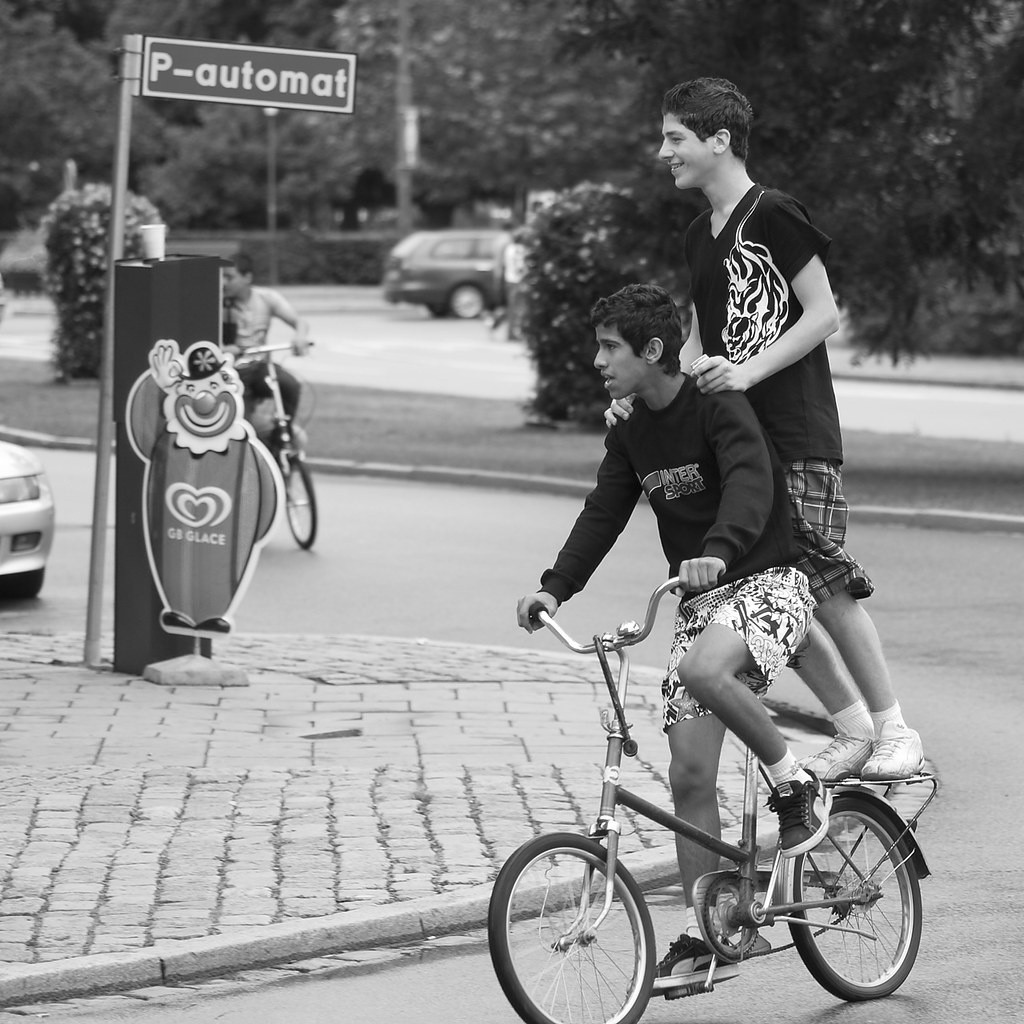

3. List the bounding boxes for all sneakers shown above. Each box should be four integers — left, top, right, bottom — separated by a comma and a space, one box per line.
765, 768, 834, 860
864, 728, 926, 780
800, 736, 874, 778
627, 932, 739, 996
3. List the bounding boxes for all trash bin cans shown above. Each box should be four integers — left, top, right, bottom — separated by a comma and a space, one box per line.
106, 252, 228, 677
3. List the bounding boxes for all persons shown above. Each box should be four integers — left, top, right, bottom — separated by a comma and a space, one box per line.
217, 252, 309, 463
516, 287, 834, 997
604, 80, 926, 781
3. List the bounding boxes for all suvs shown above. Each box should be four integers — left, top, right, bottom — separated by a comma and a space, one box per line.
383, 227, 513, 320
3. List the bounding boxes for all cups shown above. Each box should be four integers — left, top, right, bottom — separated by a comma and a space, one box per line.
139, 223, 167, 265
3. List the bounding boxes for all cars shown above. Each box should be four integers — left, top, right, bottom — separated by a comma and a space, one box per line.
0, 440, 54, 596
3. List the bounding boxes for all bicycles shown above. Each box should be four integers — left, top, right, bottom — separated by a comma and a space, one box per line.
487, 577, 939, 1024
229, 340, 319, 550
